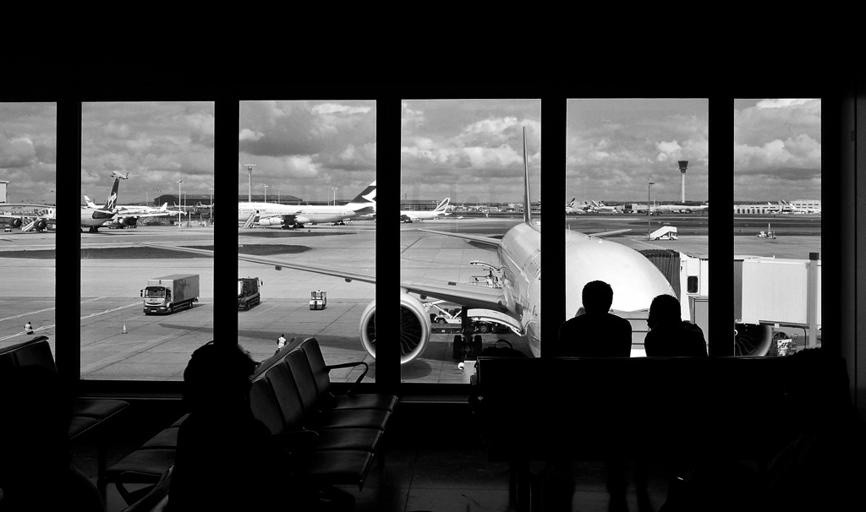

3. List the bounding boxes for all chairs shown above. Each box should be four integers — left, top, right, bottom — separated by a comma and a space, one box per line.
0, 335, 130, 512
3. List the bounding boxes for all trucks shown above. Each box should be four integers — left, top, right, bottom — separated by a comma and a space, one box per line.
138, 273, 201, 315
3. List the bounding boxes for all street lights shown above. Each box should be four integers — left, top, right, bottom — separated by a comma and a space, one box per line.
330, 185, 339, 206
176, 165, 271, 232
647, 180, 659, 244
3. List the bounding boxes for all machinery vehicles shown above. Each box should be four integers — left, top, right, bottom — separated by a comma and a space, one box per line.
649, 224, 679, 243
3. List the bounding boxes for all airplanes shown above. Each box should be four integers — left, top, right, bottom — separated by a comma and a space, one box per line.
566, 194, 708, 220
375, 197, 452, 224
132, 123, 688, 367
767, 199, 822, 216
239, 175, 379, 230
0, 169, 174, 234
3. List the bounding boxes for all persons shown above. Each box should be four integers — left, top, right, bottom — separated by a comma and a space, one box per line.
633, 294, 710, 511
23, 321, 33, 335
169, 342, 322, 511
553, 280, 632, 511
0, 365, 108, 510
276, 334, 287, 351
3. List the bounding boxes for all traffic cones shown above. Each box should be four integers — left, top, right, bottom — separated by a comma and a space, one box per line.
119, 320, 130, 335
